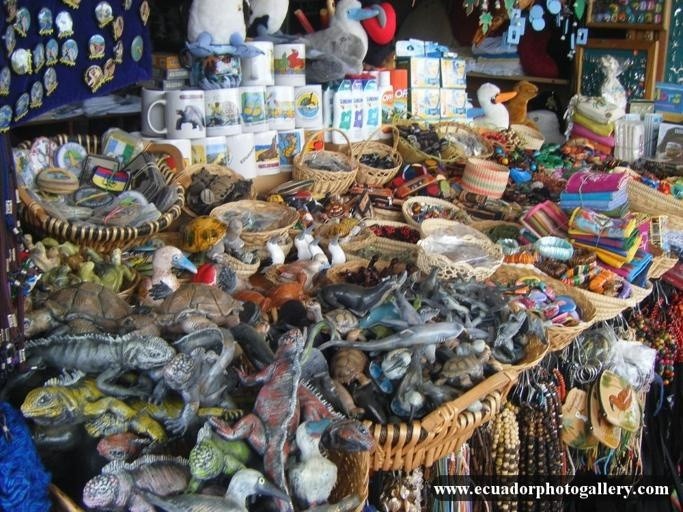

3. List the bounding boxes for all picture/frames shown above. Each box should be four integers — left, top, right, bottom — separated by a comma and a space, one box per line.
575, 0, 672, 105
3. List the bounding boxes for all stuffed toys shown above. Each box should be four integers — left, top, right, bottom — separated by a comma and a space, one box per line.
0, 217, 551, 512
181, 0, 268, 61
243, 1, 300, 46
505, 80, 543, 132
296, 1, 381, 83
466, 84, 518, 132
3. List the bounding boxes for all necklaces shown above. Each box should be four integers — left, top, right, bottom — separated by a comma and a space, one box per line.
422, 370, 573, 511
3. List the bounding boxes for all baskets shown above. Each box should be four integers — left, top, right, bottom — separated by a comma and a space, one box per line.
514, 245, 654, 322
312, 197, 536, 288
22, 132, 186, 253
644, 241, 678, 279
510, 124, 545, 152
348, 124, 403, 187
31, 261, 140, 308
626, 171, 683, 232
393, 111, 462, 165
293, 128, 359, 198
361, 326, 550, 473
48, 441, 371, 512
485, 268, 596, 352
179, 161, 299, 284
434, 121, 494, 165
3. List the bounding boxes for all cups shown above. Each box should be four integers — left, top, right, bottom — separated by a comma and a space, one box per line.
140, 41, 325, 180
